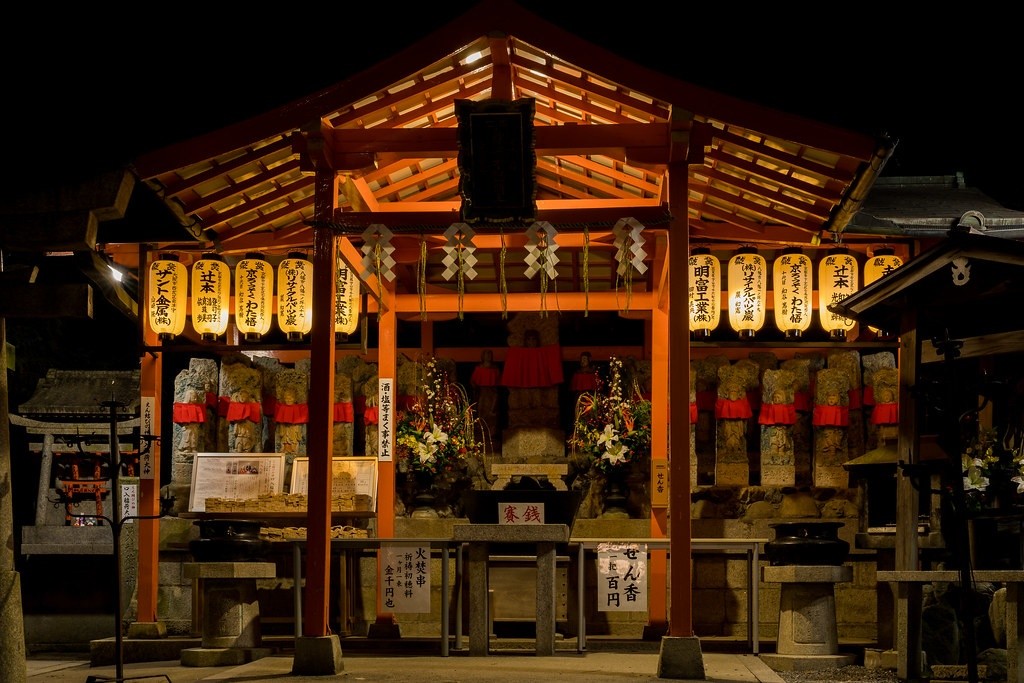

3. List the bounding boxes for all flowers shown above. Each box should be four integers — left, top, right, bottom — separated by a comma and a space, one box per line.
396, 360, 484, 483
568, 354, 651, 482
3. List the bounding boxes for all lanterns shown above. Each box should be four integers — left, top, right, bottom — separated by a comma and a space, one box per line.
278, 251, 313, 341
149, 253, 187, 341
688, 246, 720, 338
192, 253, 230, 341
728, 247, 766, 337
864, 249, 903, 337
235, 252, 273, 341
819, 247, 858, 337
335, 257, 360, 340
773, 246, 813, 338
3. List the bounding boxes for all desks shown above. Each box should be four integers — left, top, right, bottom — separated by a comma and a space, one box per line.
570, 536, 768, 653
761, 565, 853, 653
182, 562, 277, 648
288, 535, 465, 654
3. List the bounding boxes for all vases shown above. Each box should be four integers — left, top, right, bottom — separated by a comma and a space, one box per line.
606, 474, 628, 506
416, 467, 438, 507
765, 521, 852, 566
187, 519, 266, 562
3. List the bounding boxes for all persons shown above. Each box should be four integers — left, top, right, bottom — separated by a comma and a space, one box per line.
170, 329, 906, 464
